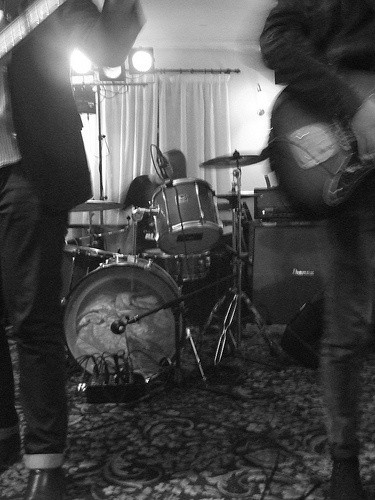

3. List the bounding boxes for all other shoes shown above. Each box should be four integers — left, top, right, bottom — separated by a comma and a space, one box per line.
332, 459, 366, 500
23, 466, 67, 500
0, 431, 21, 469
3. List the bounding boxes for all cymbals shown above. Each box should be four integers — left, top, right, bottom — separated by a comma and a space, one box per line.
199, 155, 266, 169
69, 200, 120, 212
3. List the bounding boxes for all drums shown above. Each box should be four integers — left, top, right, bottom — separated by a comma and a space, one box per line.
149, 176, 224, 256
59, 253, 187, 378
59, 243, 118, 308
142, 248, 210, 281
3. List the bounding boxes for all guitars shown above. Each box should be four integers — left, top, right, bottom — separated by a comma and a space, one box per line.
267, 67, 375, 217
0, 0, 67, 59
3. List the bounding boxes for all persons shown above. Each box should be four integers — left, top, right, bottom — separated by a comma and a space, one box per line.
119, 150, 234, 329
260, 0, 375, 500
0, 0, 147, 500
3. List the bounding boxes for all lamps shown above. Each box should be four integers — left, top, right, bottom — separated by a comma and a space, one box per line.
99, 45, 155, 81
